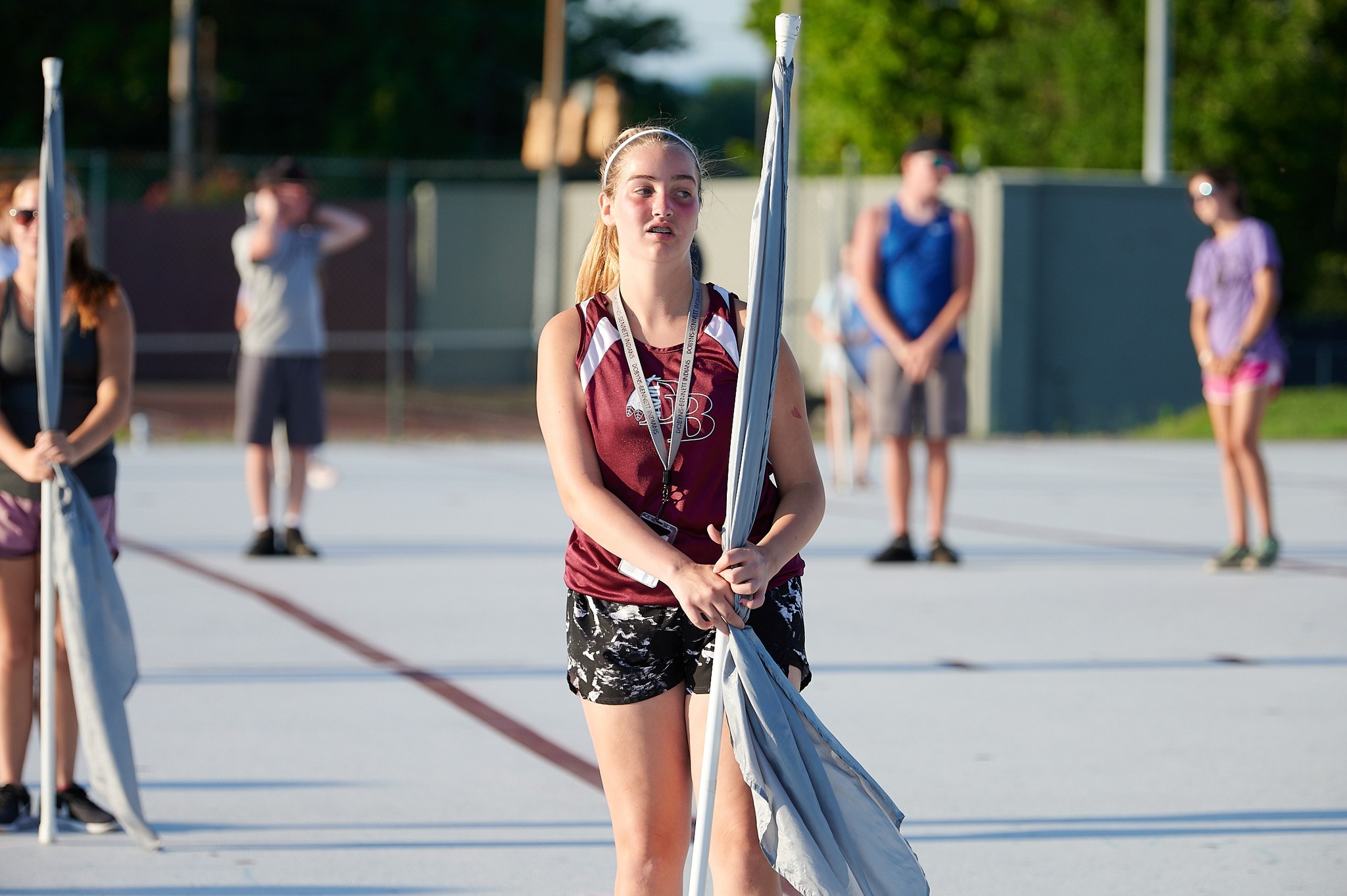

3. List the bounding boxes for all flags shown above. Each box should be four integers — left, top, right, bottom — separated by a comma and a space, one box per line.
34, 101, 166, 851
724, 56, 932, 894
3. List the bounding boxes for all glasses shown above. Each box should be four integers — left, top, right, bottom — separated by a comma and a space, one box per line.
9, 209, 75, 226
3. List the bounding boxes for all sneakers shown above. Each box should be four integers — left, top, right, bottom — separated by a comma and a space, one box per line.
56, 784, 118, 834
0, 783, 32, 830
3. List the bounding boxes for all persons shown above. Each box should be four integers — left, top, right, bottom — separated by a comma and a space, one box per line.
231, 168, 366, 557
1184, 166, 1290, 571
853, 133, 977, 565
536, 121, 827, 896
807, 236, 885, 492
0, 165, 135, 835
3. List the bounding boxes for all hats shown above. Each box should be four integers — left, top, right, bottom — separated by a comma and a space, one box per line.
909, 134, 948, 152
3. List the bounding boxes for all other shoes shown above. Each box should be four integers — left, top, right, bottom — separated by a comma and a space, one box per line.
285, 528, 317, 557
870, 534, 914, 560
246, 527, 275, 555
930, 543, 958, 563
1218, 537, 1279, 568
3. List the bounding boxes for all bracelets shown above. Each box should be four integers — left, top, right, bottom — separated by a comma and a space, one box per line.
1236, 342, 1248, 352
1199, 347, 1214, 366
